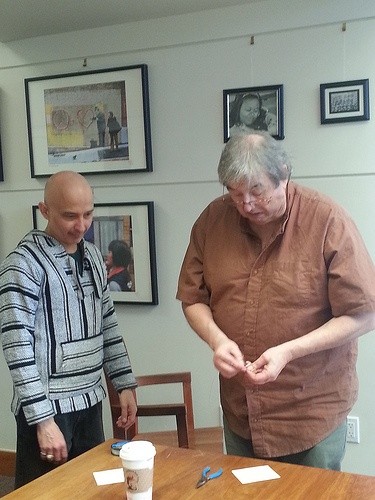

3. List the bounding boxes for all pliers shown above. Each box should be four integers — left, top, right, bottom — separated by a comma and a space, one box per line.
196, 466, 222, 488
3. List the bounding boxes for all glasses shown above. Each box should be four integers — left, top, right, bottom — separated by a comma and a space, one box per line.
222, 183, 275, 208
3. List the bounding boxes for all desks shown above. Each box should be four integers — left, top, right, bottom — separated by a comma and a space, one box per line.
0, 438, 375, 500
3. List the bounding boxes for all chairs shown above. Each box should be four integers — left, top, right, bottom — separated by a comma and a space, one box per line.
104, 336, 224, 455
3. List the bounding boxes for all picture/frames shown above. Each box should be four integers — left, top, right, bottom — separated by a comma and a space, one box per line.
32, 200, 159, 307
222, 84, 285, 143
24, 64, 153, 178
319, 78, 371, 126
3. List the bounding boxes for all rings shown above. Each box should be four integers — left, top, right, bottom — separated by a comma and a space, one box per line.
40, 452, 46, 458
46, 454, 54, 459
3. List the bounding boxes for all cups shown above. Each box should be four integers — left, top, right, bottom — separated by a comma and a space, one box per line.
119, 440, 157, 500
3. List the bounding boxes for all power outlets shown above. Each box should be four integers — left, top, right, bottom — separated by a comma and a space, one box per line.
346, 417, 360, 443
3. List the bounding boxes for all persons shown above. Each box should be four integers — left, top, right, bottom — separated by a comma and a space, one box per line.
106, 239, 134, 292
0, 171, 138, 489
176, 132, 375, 471
229, 91, 275, 134
